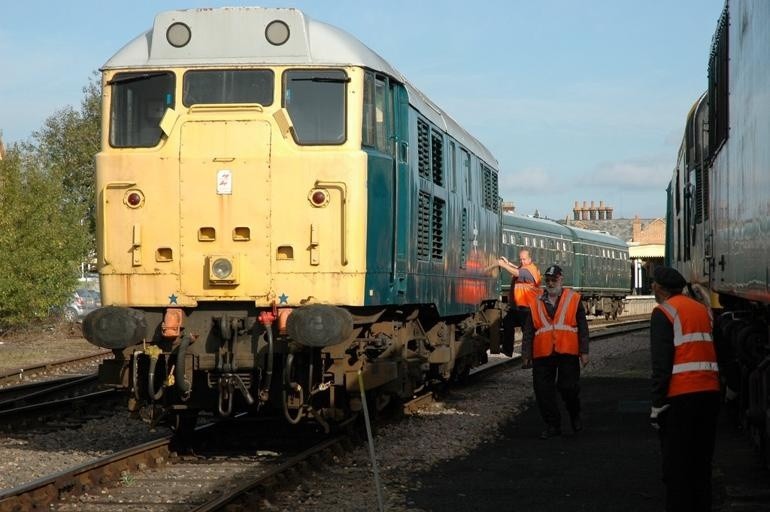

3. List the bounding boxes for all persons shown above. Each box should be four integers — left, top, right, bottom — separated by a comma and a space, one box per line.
520, 263, 592, 441
455, 247, 505, 305
497, 248, 542, 370
647, 265, 728, 511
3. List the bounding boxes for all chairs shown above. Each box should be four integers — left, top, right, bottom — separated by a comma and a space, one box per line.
571, 418, 583, 431
538, 429, 553, 440
522, 359, 530, 367
499, 347, 513, 357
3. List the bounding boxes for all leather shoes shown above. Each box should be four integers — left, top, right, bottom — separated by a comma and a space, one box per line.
650, 404, 669, 429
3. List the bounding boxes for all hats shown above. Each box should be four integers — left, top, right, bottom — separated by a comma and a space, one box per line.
48, 288, 102, 325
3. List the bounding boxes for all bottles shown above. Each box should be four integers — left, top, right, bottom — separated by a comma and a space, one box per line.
544, 266, 561, 275
654, 267, 687, 290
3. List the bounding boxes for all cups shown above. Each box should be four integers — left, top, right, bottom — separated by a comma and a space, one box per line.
546, 278, 557, 283
